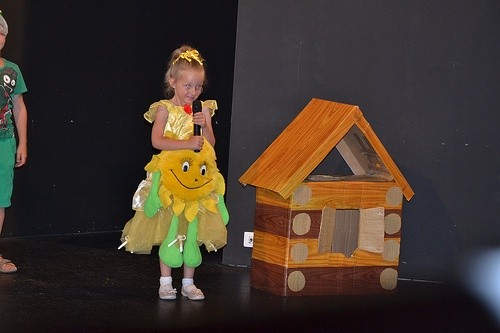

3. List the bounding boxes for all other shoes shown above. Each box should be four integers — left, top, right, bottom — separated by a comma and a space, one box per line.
181, 285, 205, 300
159, 284, 178, 299
0, 256, 17, 272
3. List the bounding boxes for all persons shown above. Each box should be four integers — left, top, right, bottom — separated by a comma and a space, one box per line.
0, 13, 28, 273
151, 45, 216, 301
